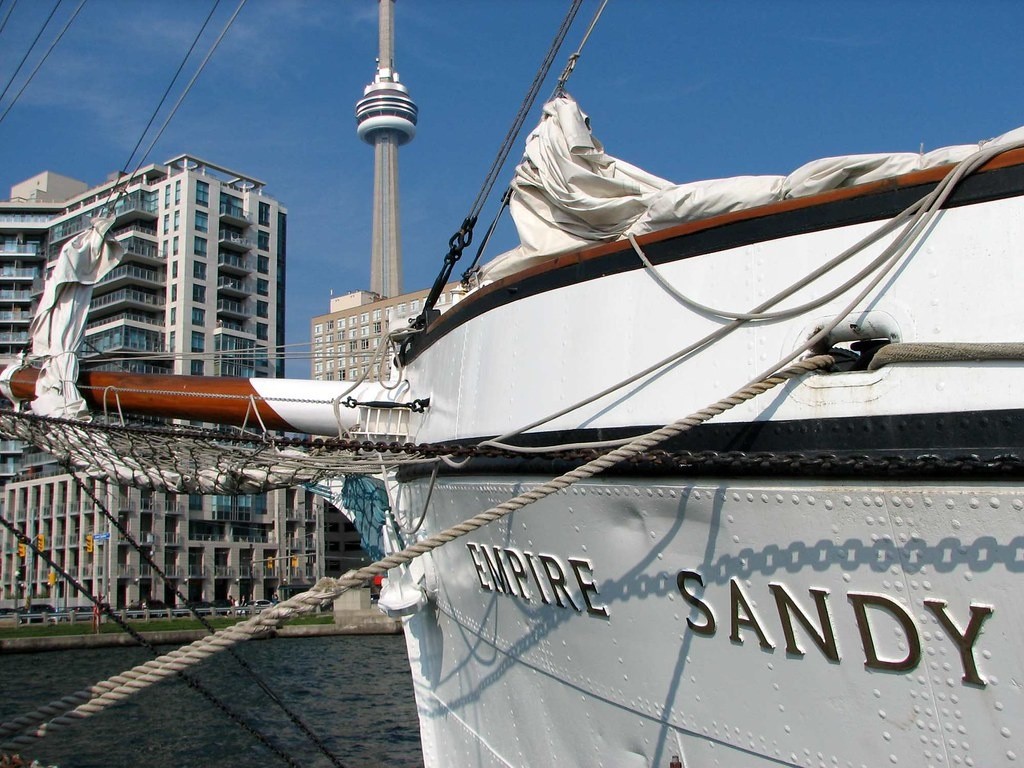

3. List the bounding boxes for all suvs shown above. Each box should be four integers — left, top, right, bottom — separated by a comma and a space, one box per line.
0, 604, 56, 624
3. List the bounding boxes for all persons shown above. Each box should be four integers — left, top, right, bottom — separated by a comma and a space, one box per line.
229, 594, 246, 607
272, 594, 278, 606
250, 594, 253, 603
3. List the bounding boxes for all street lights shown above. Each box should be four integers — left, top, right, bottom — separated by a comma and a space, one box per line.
297, 501, 325, 584
86, 487, 117, 603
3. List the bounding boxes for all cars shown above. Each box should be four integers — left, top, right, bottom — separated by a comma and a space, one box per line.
48, 606, 96, 622
128, 598, 275, 617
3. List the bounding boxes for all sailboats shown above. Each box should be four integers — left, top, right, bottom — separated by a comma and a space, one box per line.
0, 1, 1023, 768
